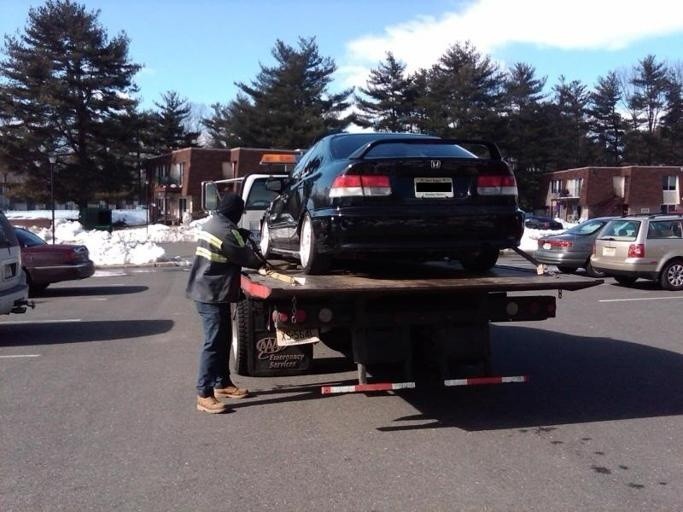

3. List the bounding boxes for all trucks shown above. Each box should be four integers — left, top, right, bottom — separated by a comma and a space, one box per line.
200, 152, 605, 397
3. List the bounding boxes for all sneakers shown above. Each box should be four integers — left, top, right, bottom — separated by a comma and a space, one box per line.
196, 393, 229, 414
214, 382, 249, 398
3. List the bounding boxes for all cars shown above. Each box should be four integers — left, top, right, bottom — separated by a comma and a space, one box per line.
260, 133, 525, 274
518, 214, 683, 292
12, 227, 95, 298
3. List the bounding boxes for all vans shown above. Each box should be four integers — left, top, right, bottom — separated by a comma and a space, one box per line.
0, 209, 35, 315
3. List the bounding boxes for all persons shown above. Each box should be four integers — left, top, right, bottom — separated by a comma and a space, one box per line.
184, 192, 267, 415
